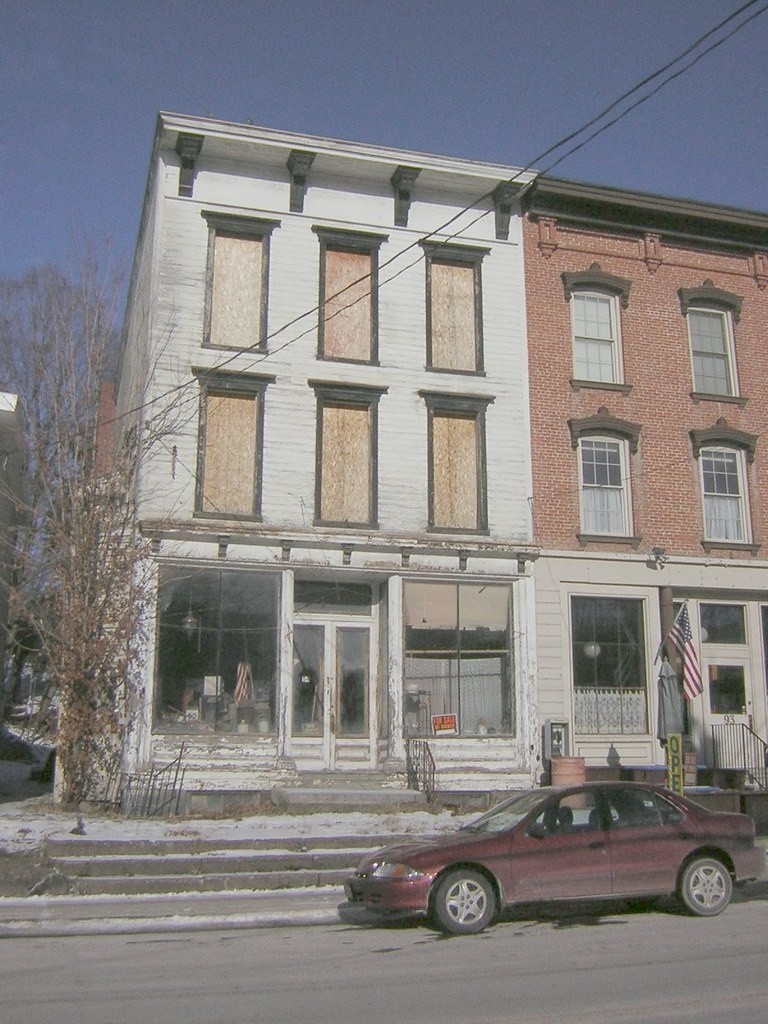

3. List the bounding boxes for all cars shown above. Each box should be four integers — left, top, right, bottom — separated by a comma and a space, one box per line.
342, 781, 767, 934
1, 723, 58, 798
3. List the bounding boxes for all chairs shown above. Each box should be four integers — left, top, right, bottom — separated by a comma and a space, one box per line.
552, 805, 574, 835
579, 809, 601, 833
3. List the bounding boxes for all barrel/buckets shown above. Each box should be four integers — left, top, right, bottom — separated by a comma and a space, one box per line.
551, 755, 587, 809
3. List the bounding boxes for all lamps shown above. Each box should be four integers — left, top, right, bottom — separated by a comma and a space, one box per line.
280, 539, 293, 551
651, 546, 668, 565
217, 535, 229, 547
342, 543, 355, 554
400, 546, 413, 557
517, 552, 530, 563
458, 548, 471, 561
151, 530, 163, 542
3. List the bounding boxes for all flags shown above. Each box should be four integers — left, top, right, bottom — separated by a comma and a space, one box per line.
669, 602, 704, 703
233, 661, 250, 705
